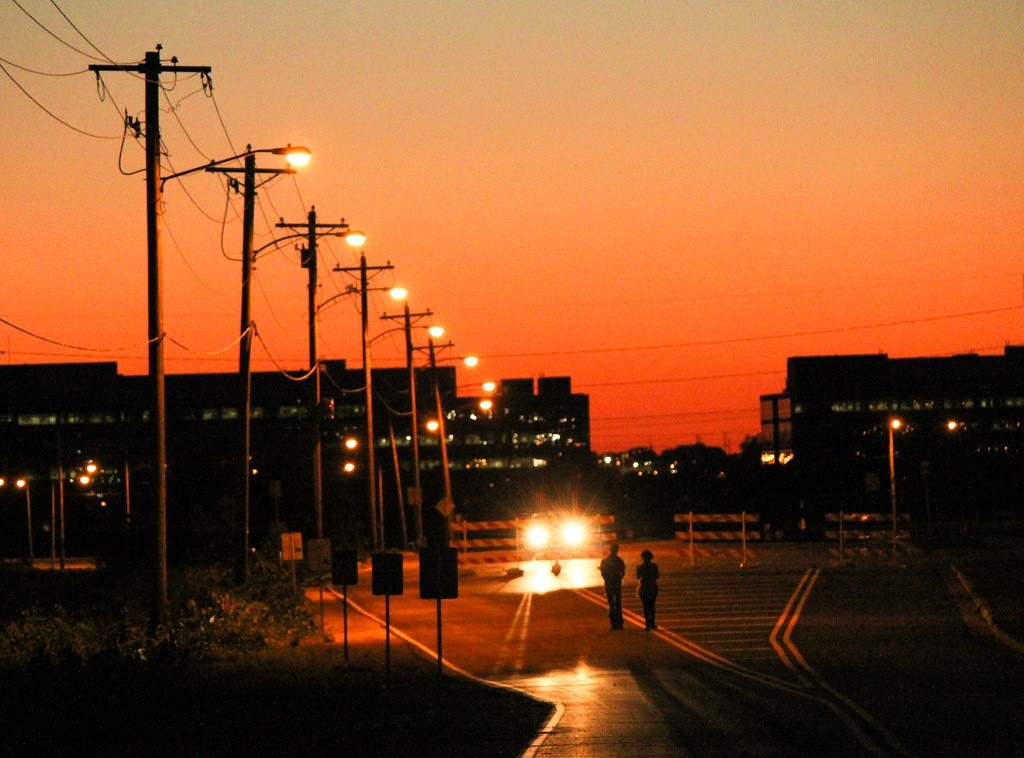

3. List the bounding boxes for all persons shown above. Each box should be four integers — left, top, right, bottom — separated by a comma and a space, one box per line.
636, 550, 660, 631
600, 544, 626, 631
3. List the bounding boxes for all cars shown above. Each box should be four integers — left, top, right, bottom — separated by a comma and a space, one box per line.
521, 509, 599, 561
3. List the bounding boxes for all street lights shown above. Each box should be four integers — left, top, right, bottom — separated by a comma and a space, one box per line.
238, 232, 369, 586
17, 479, 36, 560
157, 144, 311, 626
363, 323, 445, 556
436, 382, 498, 548
409, 342, 478, 554
888, 415, 905, 516
309, 284, 408, 537
